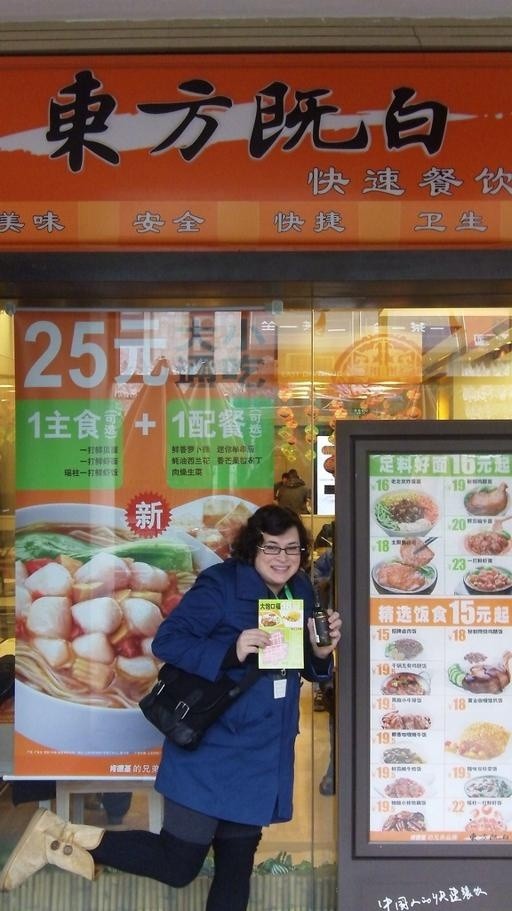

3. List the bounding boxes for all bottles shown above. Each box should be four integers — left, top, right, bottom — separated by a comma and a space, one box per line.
310, 587, 331, 647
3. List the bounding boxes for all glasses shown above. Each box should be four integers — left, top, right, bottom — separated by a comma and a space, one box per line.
256, 545, 305, 555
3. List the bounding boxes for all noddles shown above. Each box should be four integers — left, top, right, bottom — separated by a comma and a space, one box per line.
16, 523, 199, 710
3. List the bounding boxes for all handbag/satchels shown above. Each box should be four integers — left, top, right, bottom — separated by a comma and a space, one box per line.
140, 661, 242, 750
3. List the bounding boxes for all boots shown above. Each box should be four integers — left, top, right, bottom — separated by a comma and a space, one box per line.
0, 807, 107, 893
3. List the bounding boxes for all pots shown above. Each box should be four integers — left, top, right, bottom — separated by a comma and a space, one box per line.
380, 670, 432, 698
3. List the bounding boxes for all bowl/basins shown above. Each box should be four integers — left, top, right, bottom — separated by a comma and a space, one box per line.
373, 489, 511, 596
15, 493, 260, 755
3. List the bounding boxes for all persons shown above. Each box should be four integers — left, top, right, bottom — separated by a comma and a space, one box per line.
310, 548, 336, 797
278, 469, 309, 515
274, 473, 290, 501
1, 504, 342, 910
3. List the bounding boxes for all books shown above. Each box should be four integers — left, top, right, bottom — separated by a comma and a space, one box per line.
257, 600, 306, 671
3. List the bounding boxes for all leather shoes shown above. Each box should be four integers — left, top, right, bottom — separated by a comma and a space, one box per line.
320, 775, 335, 796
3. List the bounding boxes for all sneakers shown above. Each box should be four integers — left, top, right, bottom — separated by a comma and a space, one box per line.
313, 688, 326, 712
